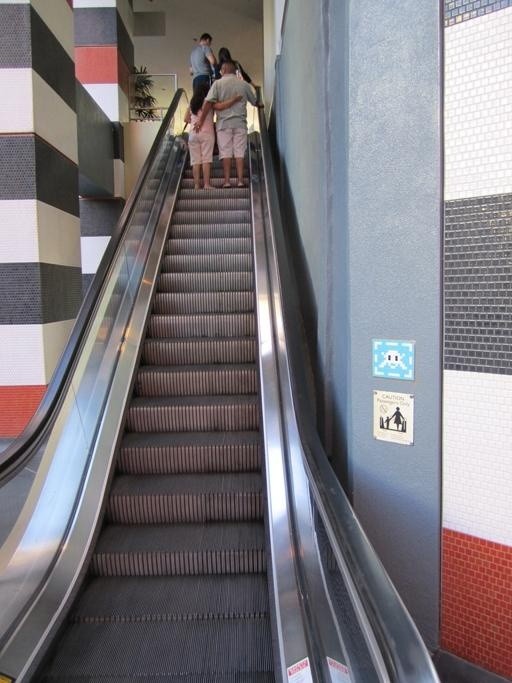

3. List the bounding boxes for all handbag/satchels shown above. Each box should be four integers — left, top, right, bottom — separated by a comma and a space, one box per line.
235, 62, 243, 81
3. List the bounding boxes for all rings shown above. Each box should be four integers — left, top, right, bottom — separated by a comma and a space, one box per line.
198, 126, 199, 128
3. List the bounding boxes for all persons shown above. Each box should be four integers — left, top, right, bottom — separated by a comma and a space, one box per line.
211, 47, 256, 88
189, 32, 217, 87
183, 79, 243, 189
192, 61, 265, 189
